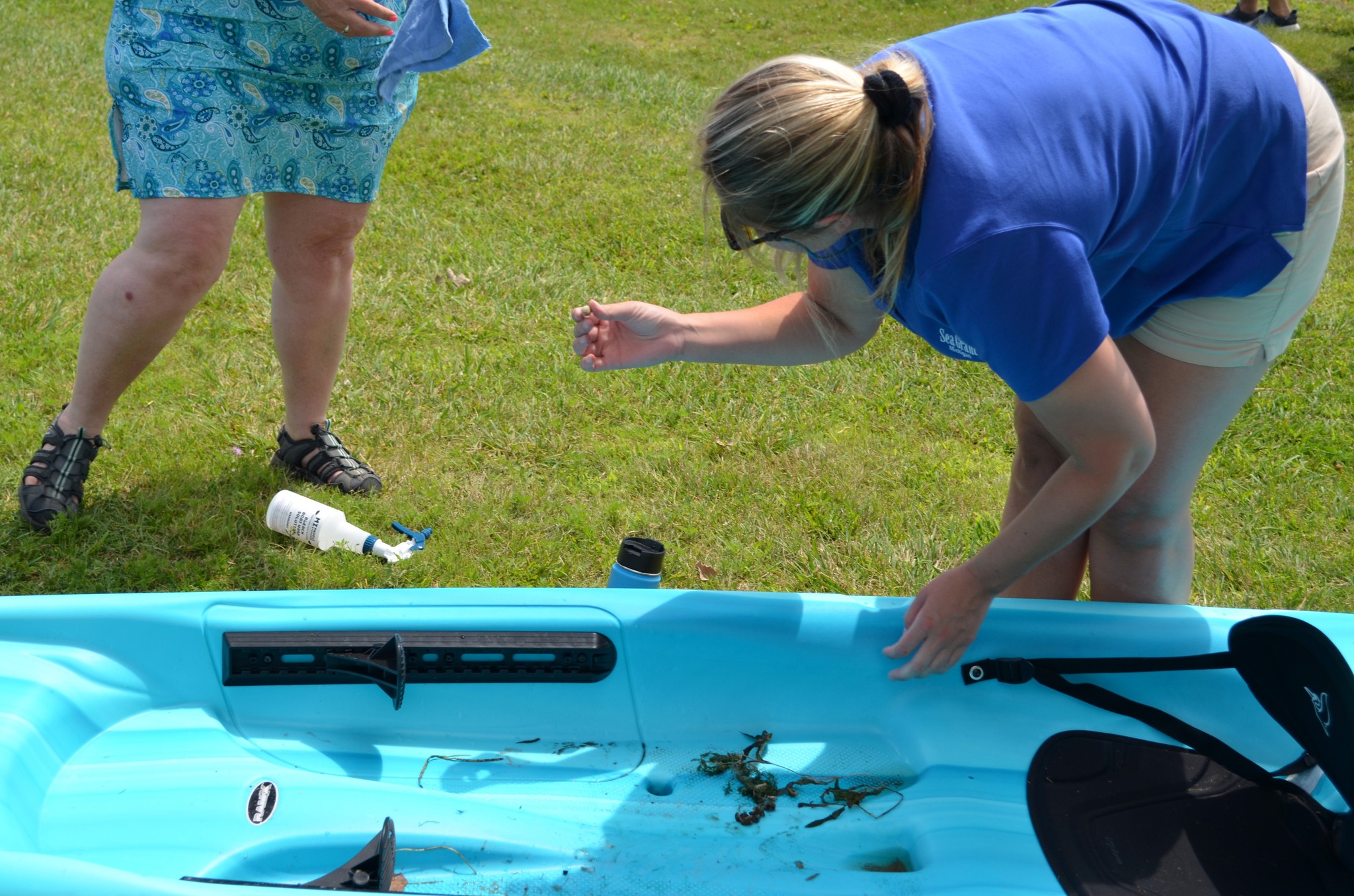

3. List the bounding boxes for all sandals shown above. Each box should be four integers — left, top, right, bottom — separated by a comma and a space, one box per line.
273, 418, 383, 498
19, 403, 111, 529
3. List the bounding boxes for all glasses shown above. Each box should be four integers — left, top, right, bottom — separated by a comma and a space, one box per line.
719, 199, 859, 252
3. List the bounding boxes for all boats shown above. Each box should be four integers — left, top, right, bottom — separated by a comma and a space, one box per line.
1, 586, 1354, 896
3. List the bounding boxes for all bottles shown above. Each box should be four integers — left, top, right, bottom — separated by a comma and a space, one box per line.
606, 534, 665, 589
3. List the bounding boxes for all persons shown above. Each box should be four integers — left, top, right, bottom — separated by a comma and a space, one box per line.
1219, 0, 1300, 31
571, 0, 1346, 679
17, 0, 420, 525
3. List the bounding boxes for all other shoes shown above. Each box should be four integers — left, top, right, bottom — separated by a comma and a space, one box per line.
1250, 6, 1300, 32
1220, 2, 1265, 26
